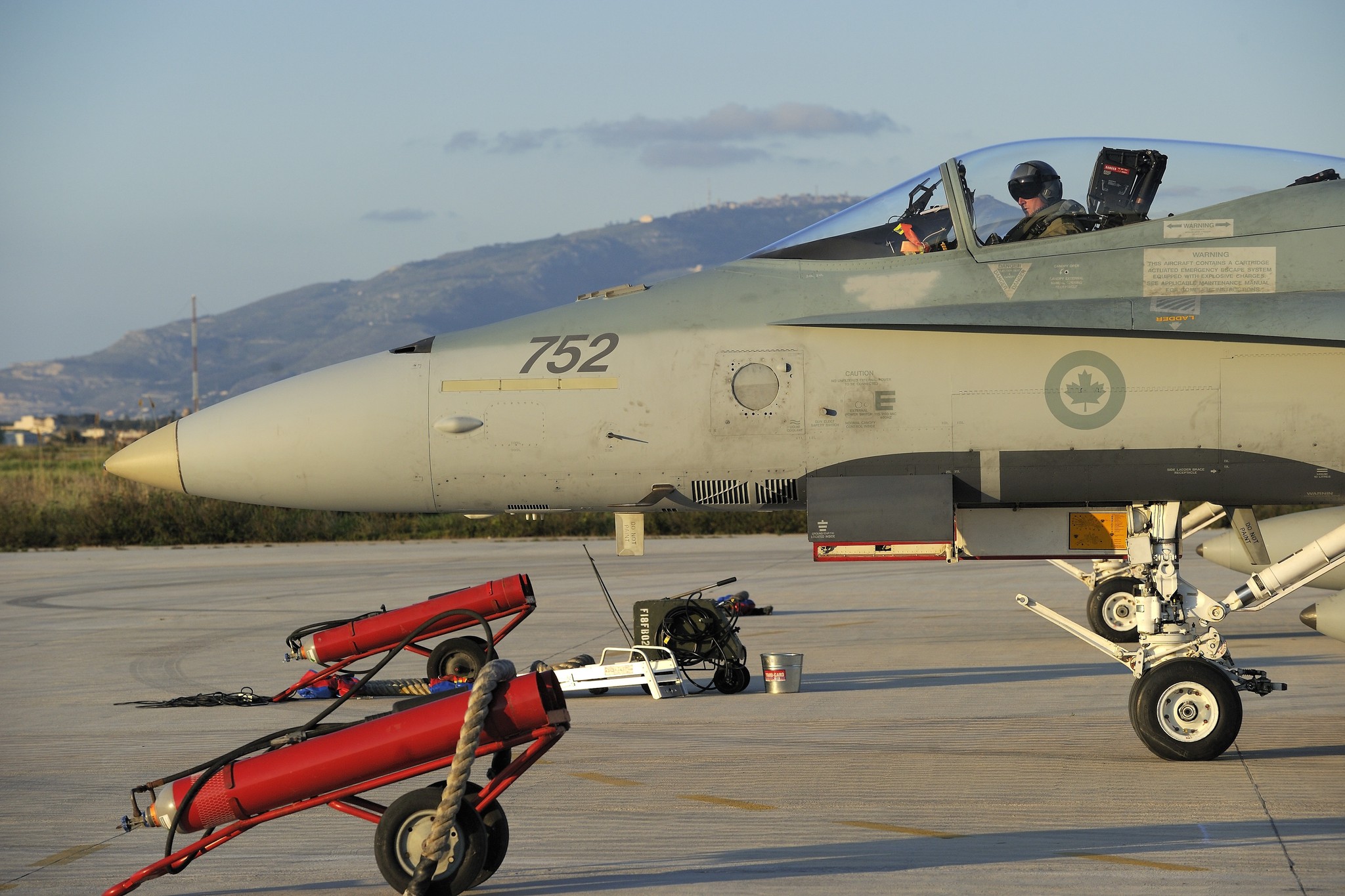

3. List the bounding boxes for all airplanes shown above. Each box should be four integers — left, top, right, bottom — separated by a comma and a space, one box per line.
103, 135, 1345, 762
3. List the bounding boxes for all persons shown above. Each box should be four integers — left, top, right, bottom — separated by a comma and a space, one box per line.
984, 159, 1089, 247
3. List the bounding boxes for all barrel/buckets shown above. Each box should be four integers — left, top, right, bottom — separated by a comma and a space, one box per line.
760, 652, 804, 694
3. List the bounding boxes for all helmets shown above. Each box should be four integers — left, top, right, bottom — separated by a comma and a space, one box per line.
1008, 160, 1062, 205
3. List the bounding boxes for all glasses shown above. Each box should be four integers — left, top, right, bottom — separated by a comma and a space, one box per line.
1008, 175, 1059, 202
